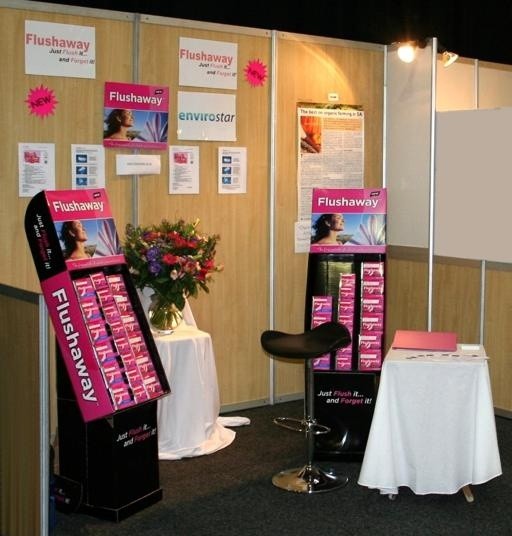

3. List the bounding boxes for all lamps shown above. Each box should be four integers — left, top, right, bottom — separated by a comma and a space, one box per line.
437, 41, 460, 67
396, 37, 427, 65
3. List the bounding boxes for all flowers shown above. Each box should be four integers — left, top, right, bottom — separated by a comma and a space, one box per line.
124, 217, 226, 328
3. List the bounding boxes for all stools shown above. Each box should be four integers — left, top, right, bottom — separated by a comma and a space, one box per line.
260, 321, 354, 494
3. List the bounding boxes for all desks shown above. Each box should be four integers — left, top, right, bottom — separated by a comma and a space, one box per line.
123, 285, 253, 460
359, 338, 503, 503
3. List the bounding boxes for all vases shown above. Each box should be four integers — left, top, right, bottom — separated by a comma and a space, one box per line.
148, 293, 184, 333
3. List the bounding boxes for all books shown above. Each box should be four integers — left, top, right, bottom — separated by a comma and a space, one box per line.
392, 330, 456, 352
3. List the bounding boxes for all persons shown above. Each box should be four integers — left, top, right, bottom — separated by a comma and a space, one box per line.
311, 213, 344, 245
104, 108, 134, 140
58, 220, 91, 261
